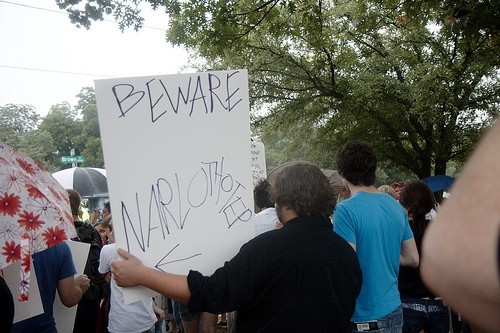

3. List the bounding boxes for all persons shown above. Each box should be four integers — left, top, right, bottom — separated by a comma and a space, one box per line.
111, 164, 363, 333
420, 116, 500, 333
377, 181, 447, 222
252, 180, 283, 235
397, 180, 453, 333
332, 141, 421, 333
0, 188, 232, 333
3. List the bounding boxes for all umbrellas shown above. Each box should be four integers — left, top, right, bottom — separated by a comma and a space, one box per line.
0, 141, 81, 302
420, 175, 455, 193
51, 162, 110, 199
